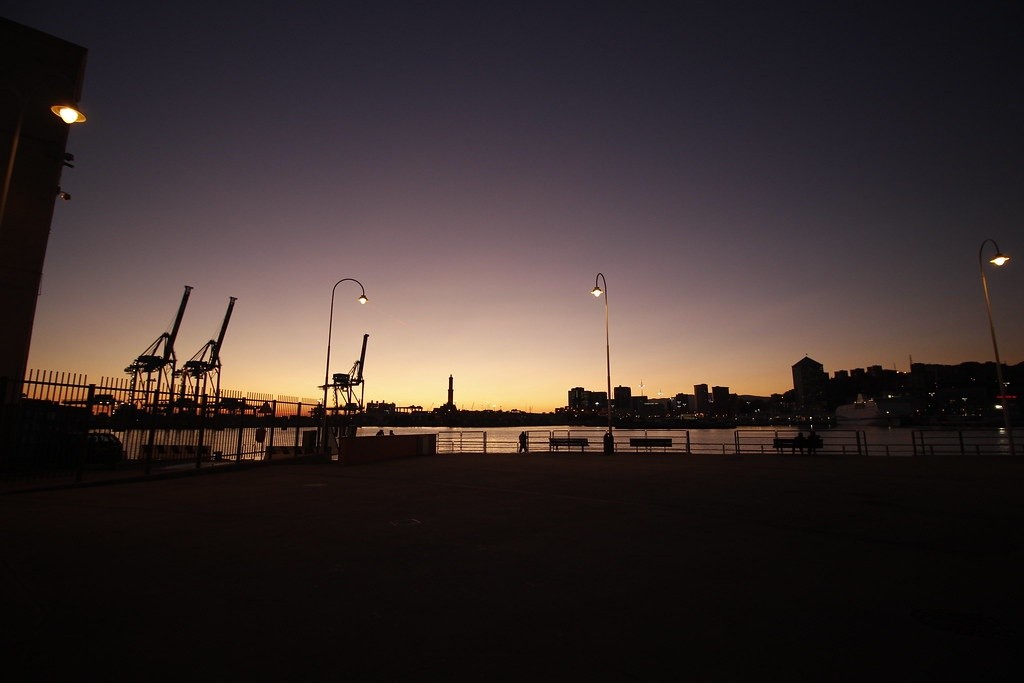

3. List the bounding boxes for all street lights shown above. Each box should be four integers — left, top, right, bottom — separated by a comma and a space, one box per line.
0, 51, 88, 225
979, 237, 1017, 457
590, 273, 612, 438
320, 277, 368, 452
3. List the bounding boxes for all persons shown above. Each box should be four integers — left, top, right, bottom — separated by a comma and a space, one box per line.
791, 431, 816, 455
376, 430, 394, 436
519, 433, 529, 455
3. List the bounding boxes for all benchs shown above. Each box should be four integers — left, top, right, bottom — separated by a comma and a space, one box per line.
773, 439, 823, 456
549, 437, 589, 453
630, 439, 672, 453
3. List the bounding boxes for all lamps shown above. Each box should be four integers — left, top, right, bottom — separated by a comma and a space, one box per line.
50, 101, 87, 124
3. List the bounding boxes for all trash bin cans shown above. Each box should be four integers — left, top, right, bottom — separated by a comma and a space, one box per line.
603, 433, 614, 456
